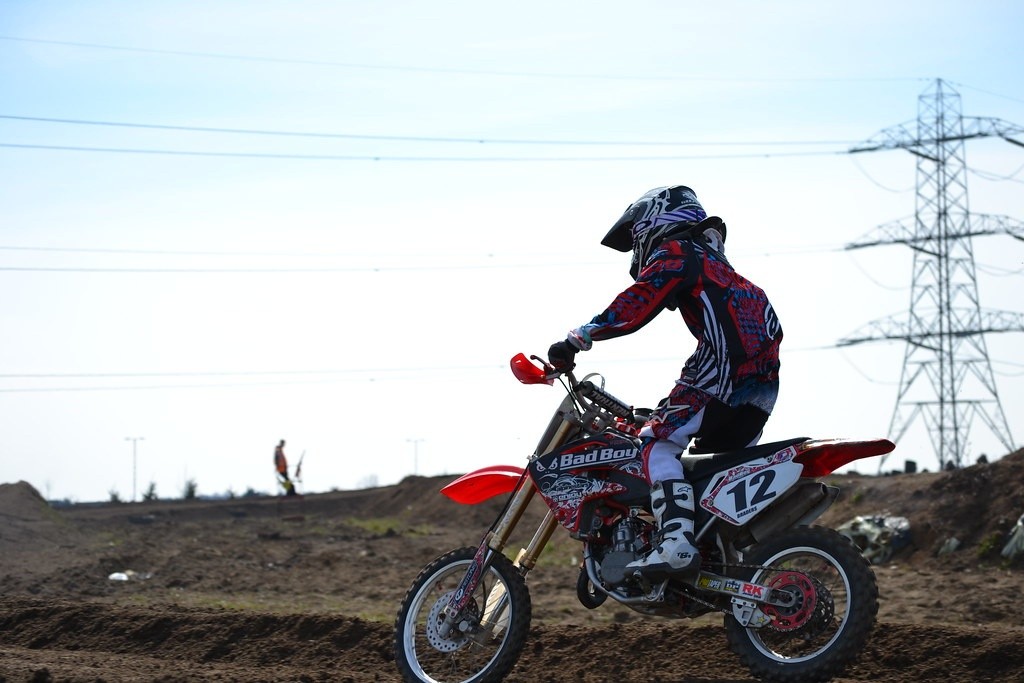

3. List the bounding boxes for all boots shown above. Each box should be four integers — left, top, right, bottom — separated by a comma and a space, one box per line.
625, 477, 702, 584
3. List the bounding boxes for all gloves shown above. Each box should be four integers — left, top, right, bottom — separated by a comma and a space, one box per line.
548, 339, 575, 373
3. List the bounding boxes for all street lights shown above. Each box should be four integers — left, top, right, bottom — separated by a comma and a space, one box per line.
125, 437, 145, 501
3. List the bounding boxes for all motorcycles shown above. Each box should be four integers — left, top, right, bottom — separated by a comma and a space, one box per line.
392, 347, 896, 683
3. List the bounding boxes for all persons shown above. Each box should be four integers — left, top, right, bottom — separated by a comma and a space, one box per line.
546, 183, 784, 581
273, 439, 305, 496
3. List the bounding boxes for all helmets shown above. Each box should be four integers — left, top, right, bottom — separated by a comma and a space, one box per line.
601, 184, 708, 282
